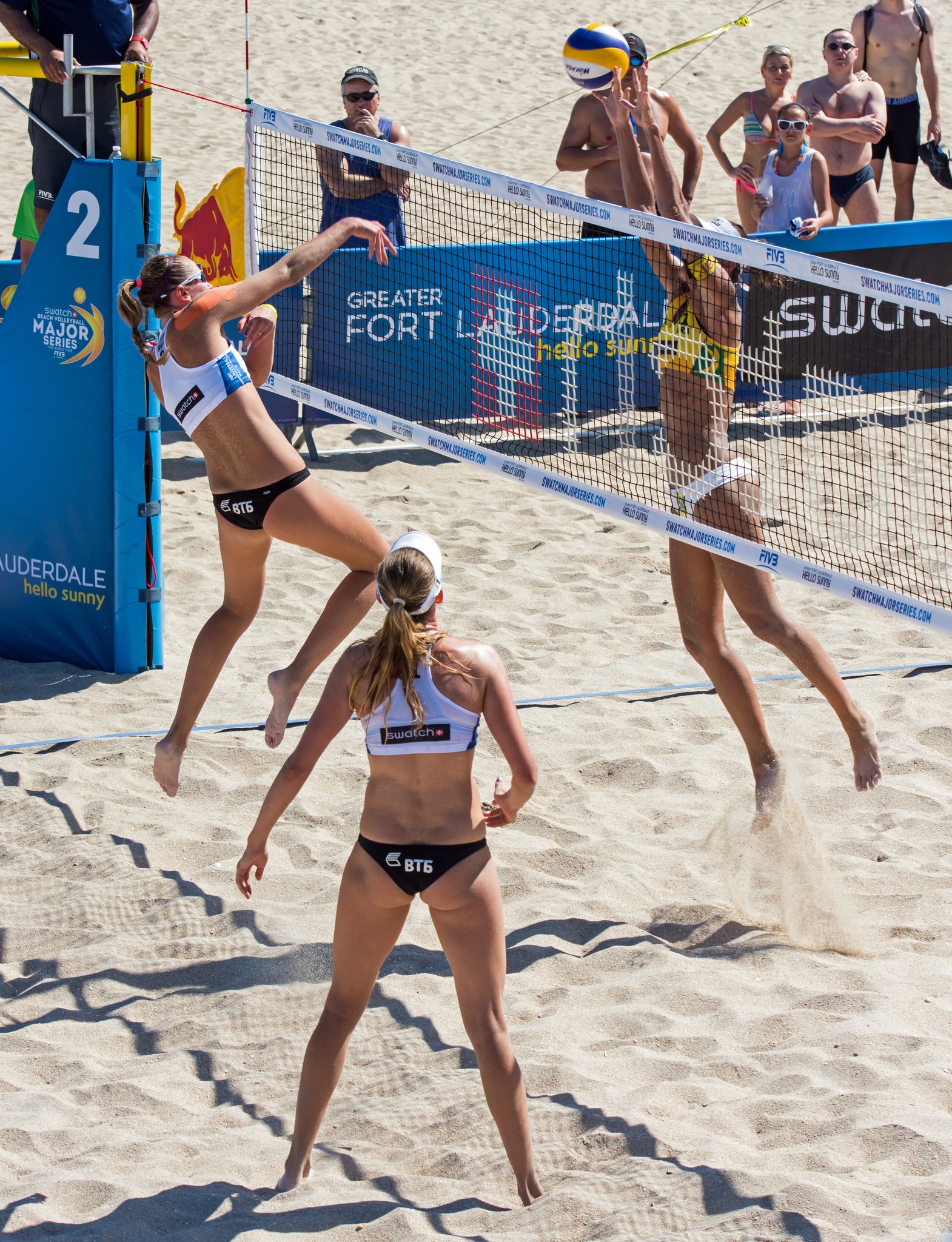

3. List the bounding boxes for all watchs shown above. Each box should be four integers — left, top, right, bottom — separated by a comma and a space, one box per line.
130, 36, 149, 50
377, 133, 387, 140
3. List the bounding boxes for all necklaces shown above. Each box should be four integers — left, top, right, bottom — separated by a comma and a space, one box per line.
826, 74, 856, 95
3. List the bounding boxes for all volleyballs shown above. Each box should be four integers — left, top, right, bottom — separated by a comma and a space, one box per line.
562, 22, 631, 93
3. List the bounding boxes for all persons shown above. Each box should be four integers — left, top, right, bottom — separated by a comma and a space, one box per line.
556, 32, 704, 417
315, 65, 411, 249
117, 217, 398, 797
234, 532, 539, 1209
0, 0, 159, 235
592, 65, 883, 834
706, 0, 941, 417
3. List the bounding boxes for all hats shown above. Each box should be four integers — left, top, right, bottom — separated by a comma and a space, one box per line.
623, 32, 647, 60
341, 66, 379, 88
688, 213, 750, 292
376, 531, 442, 616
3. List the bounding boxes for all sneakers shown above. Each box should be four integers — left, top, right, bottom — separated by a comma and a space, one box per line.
918, 140, 952, 190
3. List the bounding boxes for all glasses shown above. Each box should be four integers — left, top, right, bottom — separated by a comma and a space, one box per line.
824, 42, 856, 51
343, 91, 379, 103
777, 119, 809, 132
765, 45, 792, 54
631, 56, 645, 67
160, 270, 207, 298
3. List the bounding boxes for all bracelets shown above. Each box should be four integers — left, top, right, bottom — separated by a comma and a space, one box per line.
258, 304, 278, 320
12, 179, 40, 275
